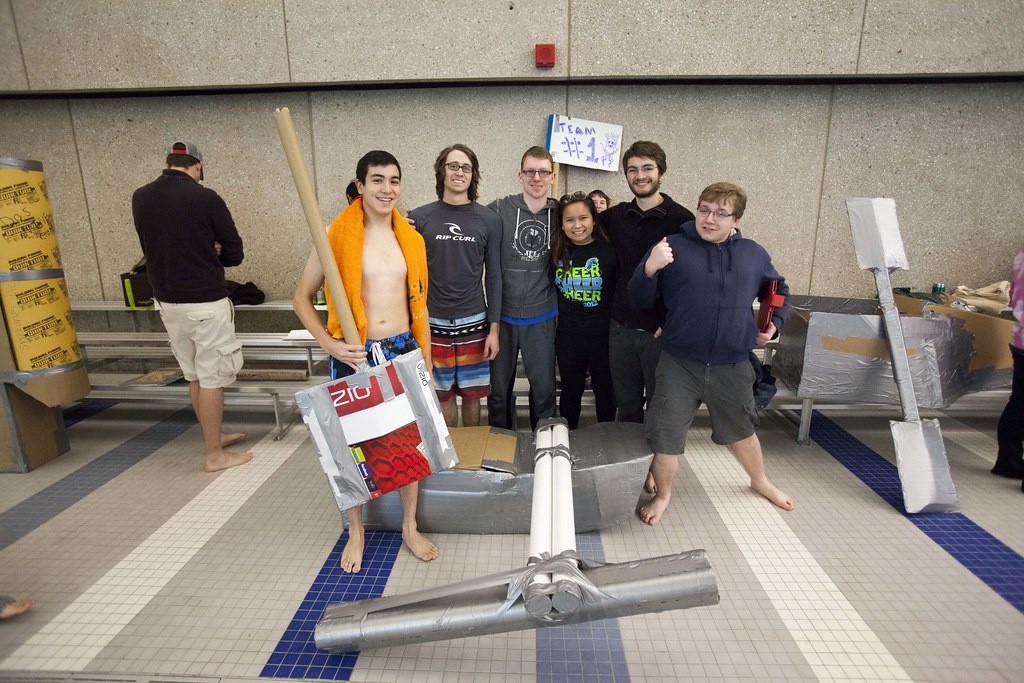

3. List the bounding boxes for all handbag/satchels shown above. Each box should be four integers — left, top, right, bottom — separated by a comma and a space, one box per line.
120, 256, 154, 308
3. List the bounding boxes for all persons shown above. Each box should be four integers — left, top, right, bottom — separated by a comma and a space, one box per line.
294, 150, 439, 573
346, 180, 361, 204
597, 139, 794, 526
409, 143, 503, 427
991, 249, 1024, 496
131, 141, 254, 472
547, 190, 615, 431
589, 189, 611, 212
404, 145, 561, 431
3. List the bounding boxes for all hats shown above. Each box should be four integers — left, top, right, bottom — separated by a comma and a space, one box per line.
169, 141, 204, 181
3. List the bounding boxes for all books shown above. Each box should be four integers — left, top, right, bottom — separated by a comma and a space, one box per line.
757, 279, 785, 332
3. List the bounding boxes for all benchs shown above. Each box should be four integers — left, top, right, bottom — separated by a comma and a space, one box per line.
67, 300, 331, 442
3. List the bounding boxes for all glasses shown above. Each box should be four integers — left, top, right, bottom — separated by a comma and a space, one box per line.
443, 162, 472, 174
559, 190, 587, 206
625, 164, 660, 175
696, 206, 735, 221
522, 167, 553, 179
